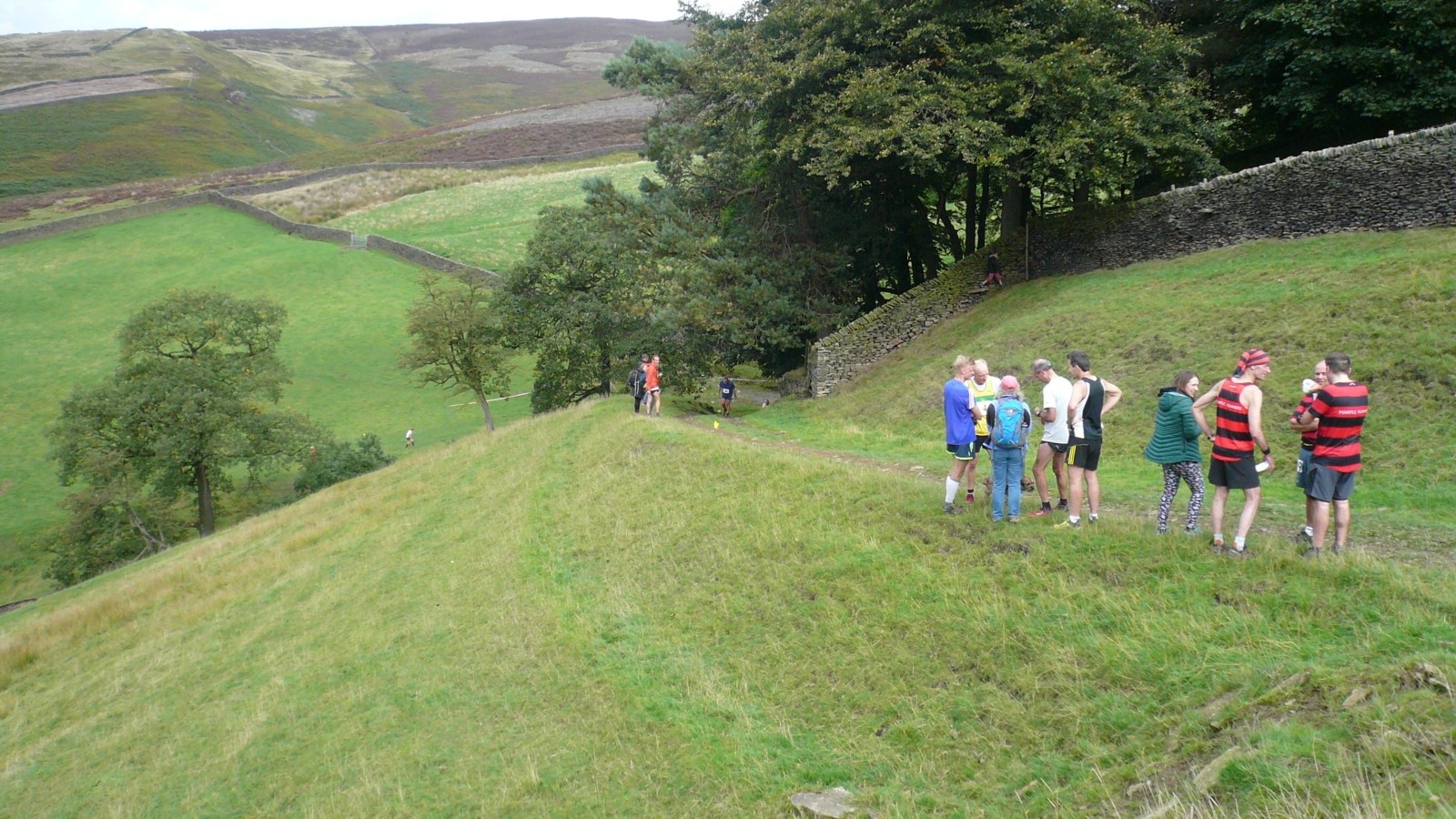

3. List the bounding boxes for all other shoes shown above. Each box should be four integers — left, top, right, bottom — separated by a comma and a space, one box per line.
1027, 508, 1052, 517
992, 517, 1002, 522
1051, 506, 1068, 512
1157, 530, 1166, 535
1299, 549, 1323, 558
1009, 516, 1019, 522
722, 409, 729, 417
1327, 547, 1343, 555
1288, 532, 1313, 544
1213, 543, 1227, 553
1228, 545, 1253, 558
656, 414, 660, 418
966, 494, 974, 503
1186, 528, 1201, 534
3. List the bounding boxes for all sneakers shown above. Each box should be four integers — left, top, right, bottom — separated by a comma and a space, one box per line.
942, 502, 963, 515
1053, 520, 1081, 530
1090, 517, 1099, 525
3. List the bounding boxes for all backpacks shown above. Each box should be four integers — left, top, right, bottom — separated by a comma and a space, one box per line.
991, 398, 1026, 448
627, 370, 642, 387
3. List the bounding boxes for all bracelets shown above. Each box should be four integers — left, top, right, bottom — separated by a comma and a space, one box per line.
1206, 434, 1215, 440
1036, 411, 1041, 417
1261, 446, 1271, 455
1297, 414, 1303, 421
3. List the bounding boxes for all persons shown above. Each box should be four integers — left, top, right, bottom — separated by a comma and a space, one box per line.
986, 374, 1033, 524
1053, 350, 1122, 529
1027, 358, 1074, 517
1156, 370, 1205, 536
405, 428, 416, 448
1191, 348, 1274, 559
629, 353, 664, 418
964, 359, 1003, 505
980, 251, 1004, 289
717, 376, 737, 417
941, 355, 982, 516
1291, 353, 1368, 556
